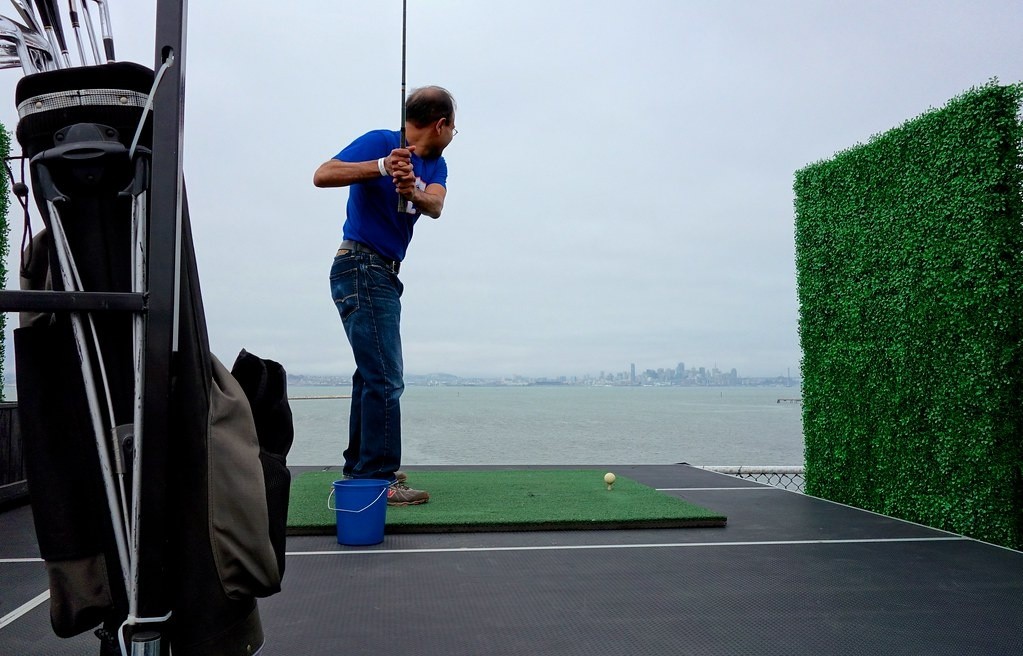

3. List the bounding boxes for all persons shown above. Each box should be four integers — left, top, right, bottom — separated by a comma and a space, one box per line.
313, 85, 458, 506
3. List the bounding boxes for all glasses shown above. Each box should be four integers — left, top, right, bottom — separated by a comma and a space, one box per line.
447, 122, 458, 136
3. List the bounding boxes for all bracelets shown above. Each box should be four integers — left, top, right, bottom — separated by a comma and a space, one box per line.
377, 158, 388, 176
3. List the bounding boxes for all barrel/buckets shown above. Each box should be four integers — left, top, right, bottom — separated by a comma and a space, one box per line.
328, 479, 392, 546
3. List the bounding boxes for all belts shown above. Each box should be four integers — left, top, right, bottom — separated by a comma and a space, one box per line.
338, 240, 398, 272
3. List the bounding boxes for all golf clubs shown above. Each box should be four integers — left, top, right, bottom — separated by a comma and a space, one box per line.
398, 2, 407, 209
0, 1, 117, 77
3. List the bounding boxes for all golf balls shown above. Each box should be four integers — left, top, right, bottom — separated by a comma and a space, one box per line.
604, 472, 616, 484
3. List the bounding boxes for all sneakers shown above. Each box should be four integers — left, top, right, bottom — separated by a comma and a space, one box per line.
388, 482, 429, 506
395, 470, 406, 481
